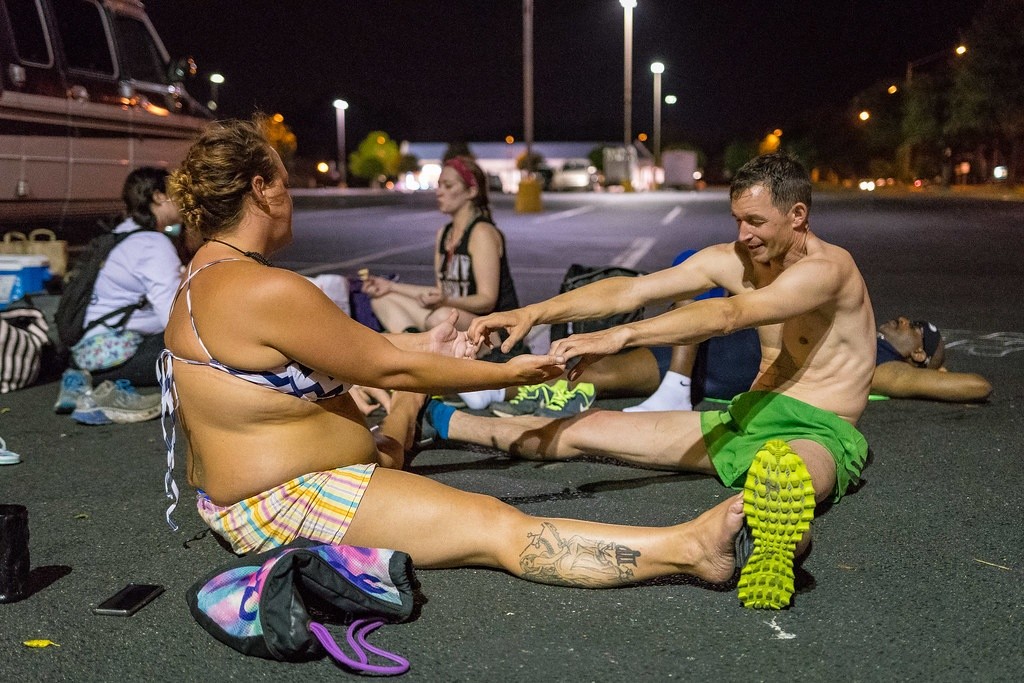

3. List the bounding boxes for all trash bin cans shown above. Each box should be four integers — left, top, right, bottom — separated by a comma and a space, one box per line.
515, 180, 541, 213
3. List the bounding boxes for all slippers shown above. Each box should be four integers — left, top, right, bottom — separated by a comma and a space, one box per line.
1, 437, 20, 464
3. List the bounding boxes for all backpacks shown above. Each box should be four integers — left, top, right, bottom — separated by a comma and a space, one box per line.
549, 263, 648, 370
55, 228, 168, 346
187, 534, 422, 675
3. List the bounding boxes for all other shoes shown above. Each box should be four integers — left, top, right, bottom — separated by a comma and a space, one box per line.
739, 440, 818, 610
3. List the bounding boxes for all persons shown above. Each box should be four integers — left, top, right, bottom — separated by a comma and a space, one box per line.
413, 152, 876, 610
458, 250, 989, 413
362, 155, 524, 359
155, 118, 746, 588
71, 166, 184, 387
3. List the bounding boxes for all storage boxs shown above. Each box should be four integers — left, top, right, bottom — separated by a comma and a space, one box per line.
0, 255, 51, 307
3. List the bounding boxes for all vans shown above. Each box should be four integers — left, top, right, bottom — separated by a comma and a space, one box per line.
0, 1, 226, 237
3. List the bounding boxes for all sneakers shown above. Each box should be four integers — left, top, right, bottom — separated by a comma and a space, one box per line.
71, 379, 164, 425
489, 379, 568, 419
54, 368, 92, 412
531, 382, 597, 422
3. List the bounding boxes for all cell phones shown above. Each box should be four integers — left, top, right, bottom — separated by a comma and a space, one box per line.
92, 583, 165, 616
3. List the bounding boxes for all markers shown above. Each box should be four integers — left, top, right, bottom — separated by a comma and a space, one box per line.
868, 394, 890, 400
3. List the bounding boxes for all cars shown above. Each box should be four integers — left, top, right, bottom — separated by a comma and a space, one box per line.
538, 157, 600, 194
408, 153, 522, 196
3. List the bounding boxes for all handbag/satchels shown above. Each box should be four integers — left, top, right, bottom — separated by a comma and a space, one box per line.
1, 308, 50, 394
73, 329, 145, 371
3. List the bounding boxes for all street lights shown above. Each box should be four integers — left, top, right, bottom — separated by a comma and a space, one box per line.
650, 61, 665, 167
618, 0, 639, 146
209, 73, 225, 117
333, 99, 349, 187
